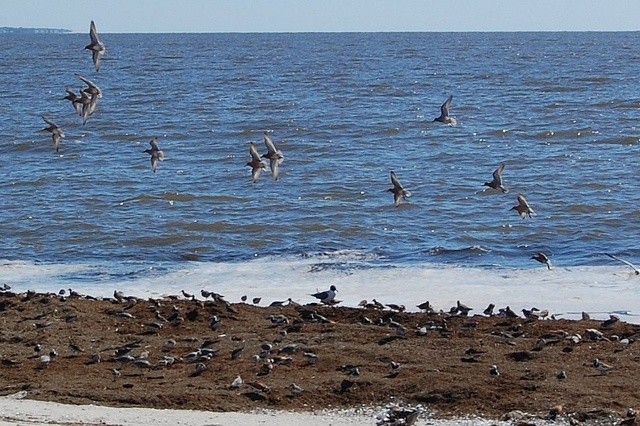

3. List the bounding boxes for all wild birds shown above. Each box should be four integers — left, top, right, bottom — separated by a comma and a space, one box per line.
260, 134, 283, 181
431, 95, 456, 127
64, 90, 83, 115
41, 116, 65, 153
76, 74, 101, 116
603, 252, 640, 274
75, 90, 91, 124
0, 284, 406, 395
80, 20, 105, 73
244, 143, 267, 183
481, 164, 509, 193
142, 136, 164, 173
386, 171, 411, 208
530, 252, 551, 270
415, 300, 634, 378
547, 402, 567, 416
508, 195, 536, 221
